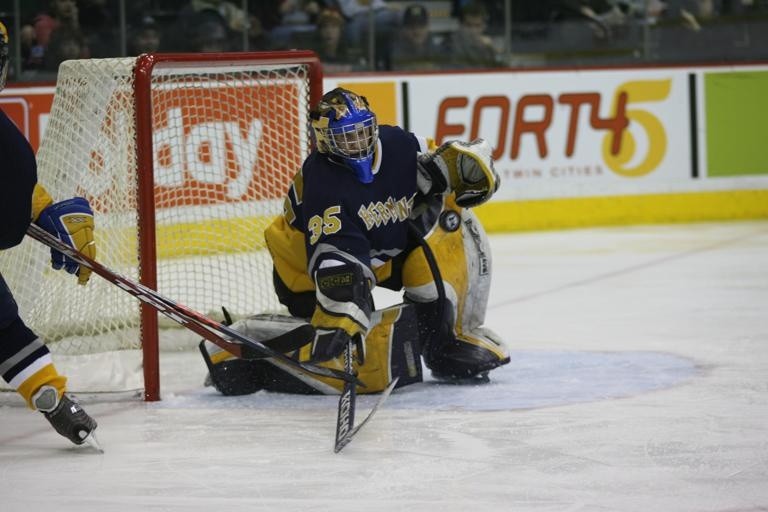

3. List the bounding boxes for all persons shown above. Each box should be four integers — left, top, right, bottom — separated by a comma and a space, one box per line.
1, 2, 766, 79
0, 26, 97, 444
201, 88, 511, 397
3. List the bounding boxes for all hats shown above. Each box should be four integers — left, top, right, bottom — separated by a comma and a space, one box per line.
315, 6, 345, 27
402, 5, 429, 28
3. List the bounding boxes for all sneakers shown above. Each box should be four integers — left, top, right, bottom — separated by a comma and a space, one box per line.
43, 390, 98, 446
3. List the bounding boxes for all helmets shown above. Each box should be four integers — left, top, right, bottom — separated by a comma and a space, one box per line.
304, 87, 382, 187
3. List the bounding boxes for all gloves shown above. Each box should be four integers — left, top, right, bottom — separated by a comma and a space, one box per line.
32, 196, 97, 287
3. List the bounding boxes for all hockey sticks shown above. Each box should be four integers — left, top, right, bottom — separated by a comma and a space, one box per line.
29, 224, 366, 388
335, 260, 400, 454
27, 225, 316, 359
27, 224, 400, 454
